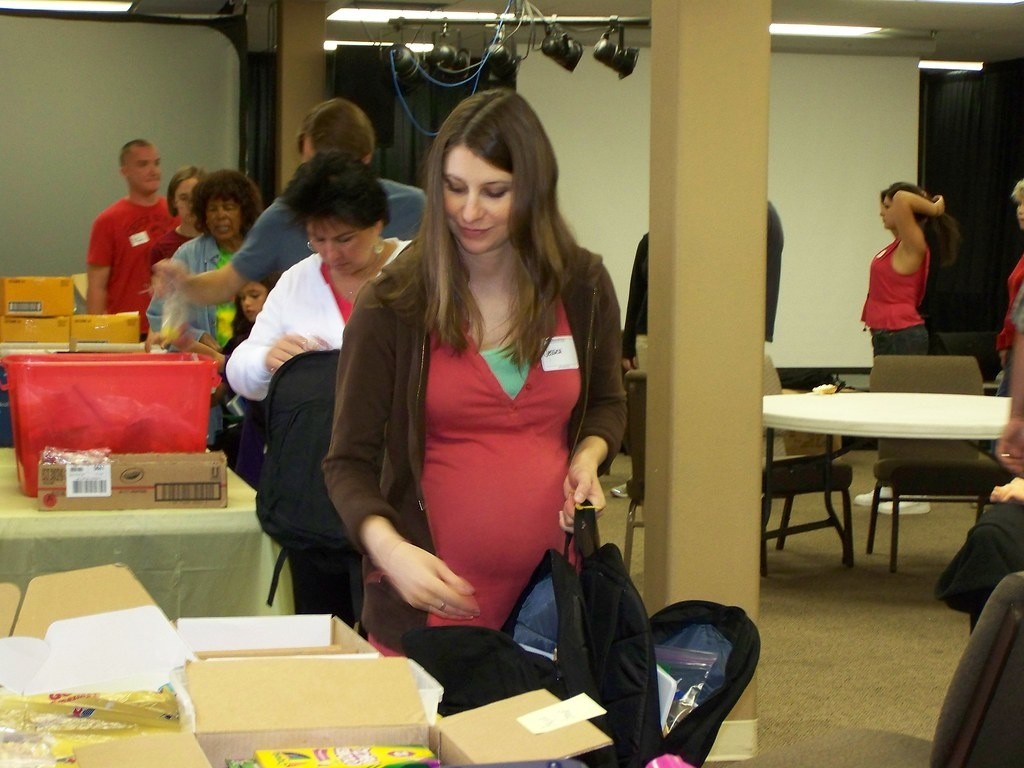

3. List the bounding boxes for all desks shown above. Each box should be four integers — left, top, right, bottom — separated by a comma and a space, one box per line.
1, 447, 286, 621
762, 392, 1011, 567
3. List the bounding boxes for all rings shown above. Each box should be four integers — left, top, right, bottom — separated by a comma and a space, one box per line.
439, 602, 446, 611
1002, 454, 1010, 456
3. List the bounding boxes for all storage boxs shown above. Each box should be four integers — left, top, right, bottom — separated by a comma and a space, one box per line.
2, 564, 615, 768
0, 276, 228, 509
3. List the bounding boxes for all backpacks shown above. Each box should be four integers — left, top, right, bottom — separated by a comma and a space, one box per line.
404, 496, 761, 768
255, 348, 354, 557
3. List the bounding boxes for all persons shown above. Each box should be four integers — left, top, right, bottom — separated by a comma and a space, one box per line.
147, 165, 272, 469
854, 182, 960, 514
223, 148, 413, 633
989, 177, 1024, 479
153, 96, 425, 311
86, 138, 174, 343
611, 201, 783, 500
322, 89, 628, 660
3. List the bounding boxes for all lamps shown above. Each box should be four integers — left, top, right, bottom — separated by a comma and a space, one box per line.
384, 14, 641, 94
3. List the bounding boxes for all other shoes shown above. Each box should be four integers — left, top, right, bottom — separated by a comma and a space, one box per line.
611, 483, 630, 498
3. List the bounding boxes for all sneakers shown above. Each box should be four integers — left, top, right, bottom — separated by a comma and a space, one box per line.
854, 486, 892, 506
877, 495, 931, 514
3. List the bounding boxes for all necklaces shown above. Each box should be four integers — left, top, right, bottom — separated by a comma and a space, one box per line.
343, 269, 368, 299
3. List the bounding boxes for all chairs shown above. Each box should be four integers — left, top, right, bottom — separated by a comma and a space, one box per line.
760, 331, 998, 576
622, 369, 647, 575
